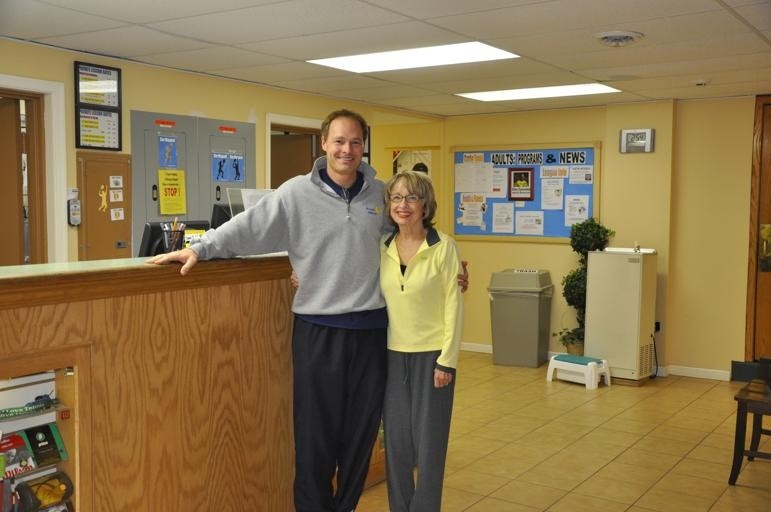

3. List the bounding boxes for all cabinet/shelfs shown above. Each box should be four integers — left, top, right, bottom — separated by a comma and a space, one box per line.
0, 339, 89, 511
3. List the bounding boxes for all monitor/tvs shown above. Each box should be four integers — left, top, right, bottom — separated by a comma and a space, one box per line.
139, 220, 209, 257
211, 204, 244, 230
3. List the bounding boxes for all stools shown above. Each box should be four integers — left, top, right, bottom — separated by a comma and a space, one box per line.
726, 376, 769, 488
545, 352, 611, 391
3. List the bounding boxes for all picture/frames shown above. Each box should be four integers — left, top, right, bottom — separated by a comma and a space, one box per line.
74, 103, 123, 152
71, 59, 122, 112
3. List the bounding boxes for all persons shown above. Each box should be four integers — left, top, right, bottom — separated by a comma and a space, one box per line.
514, 174, 528, 187
290, 169, 465, 512
147, 108, 469, 511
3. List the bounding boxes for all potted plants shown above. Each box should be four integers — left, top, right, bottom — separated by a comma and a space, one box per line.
549, 216, 614, 355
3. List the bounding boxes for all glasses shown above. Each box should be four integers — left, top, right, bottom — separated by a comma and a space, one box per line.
389, 193, 424, 203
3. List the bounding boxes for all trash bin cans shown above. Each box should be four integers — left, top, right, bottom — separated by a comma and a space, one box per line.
486, 268, 554, 368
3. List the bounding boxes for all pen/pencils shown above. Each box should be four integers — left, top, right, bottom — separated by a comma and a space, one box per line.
160, 216, 186, 253
